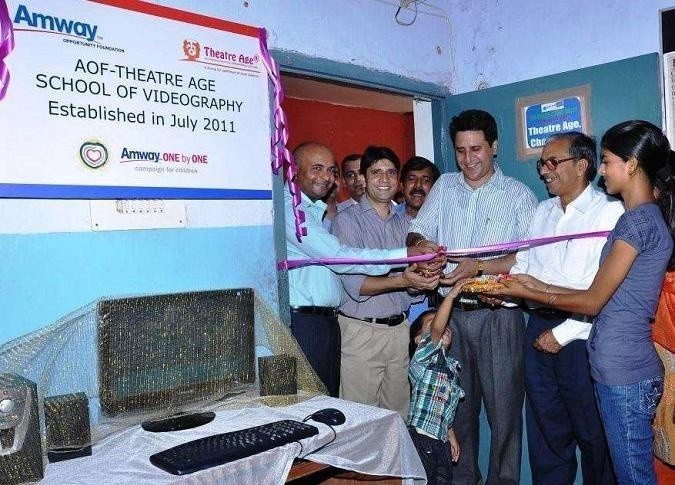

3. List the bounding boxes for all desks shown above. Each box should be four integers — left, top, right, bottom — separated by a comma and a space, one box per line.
0, 387, 402, 485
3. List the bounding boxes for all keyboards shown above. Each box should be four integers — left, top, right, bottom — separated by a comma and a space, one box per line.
150, 418, 319, 478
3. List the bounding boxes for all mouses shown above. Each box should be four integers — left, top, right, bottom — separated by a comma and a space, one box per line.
309, 406, 347, 426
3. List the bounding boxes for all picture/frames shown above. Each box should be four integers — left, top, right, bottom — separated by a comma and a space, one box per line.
515, 83, 593, 163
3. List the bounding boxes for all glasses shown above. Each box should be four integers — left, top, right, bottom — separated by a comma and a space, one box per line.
537, 157, 582, 171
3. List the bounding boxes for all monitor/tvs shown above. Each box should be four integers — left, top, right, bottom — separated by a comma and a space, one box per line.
97, 286, 258, 433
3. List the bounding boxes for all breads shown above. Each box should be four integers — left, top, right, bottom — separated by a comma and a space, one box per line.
463, 280, 506, 293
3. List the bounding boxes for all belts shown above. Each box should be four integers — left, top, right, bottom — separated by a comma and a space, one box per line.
292, 305, 336, 317
338, 310, 409, 326
530, 306, 571, 321
441, 300, 499, 314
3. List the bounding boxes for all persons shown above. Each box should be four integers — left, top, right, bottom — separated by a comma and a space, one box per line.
408, 110, 540, 485
404, 277, 475, 485
479, 131, 626, 485
485, 120, 675, 485
283, 142, 442, 422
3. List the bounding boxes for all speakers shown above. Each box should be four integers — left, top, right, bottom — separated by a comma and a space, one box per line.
42, 391, 93, 464
257, 354, 298, 397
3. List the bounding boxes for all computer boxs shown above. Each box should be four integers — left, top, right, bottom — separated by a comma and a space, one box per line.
0, 370, 43, 485
